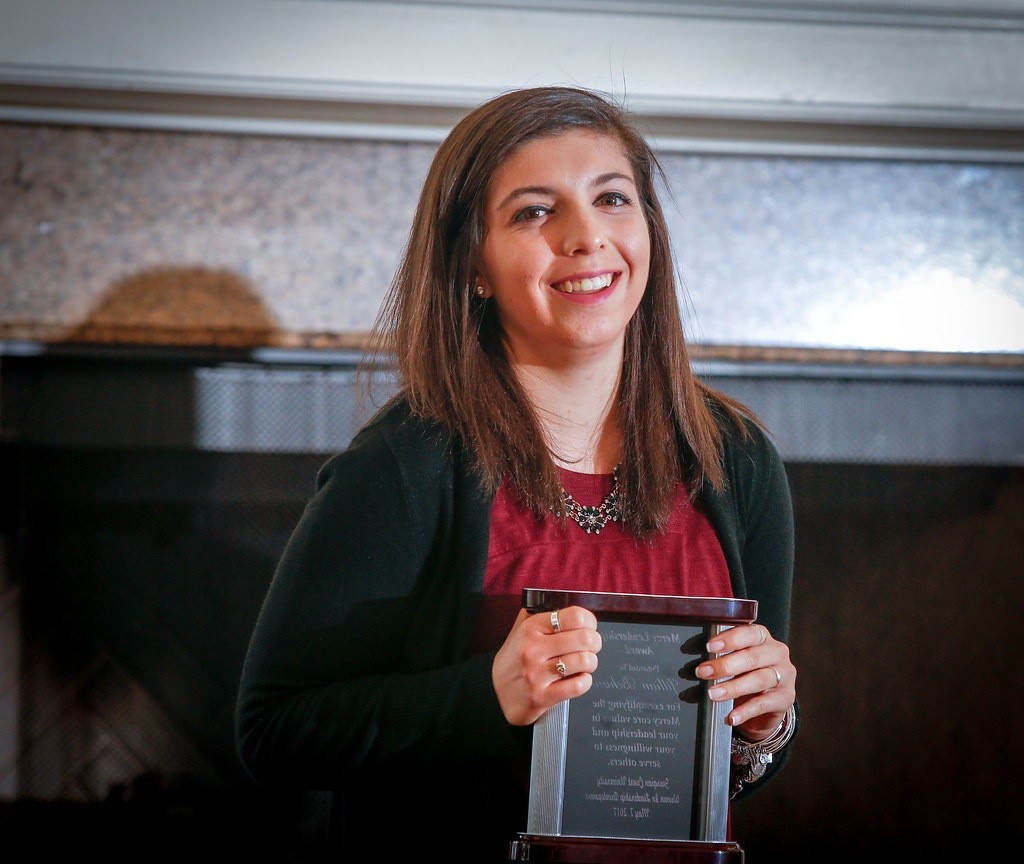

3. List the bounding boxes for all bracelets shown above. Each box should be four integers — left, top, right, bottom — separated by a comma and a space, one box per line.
731, 704, 796, 802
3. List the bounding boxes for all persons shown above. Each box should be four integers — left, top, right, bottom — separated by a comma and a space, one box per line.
230, 91, 794, 864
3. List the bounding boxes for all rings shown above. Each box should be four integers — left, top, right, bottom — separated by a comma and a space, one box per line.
751, 623, 765, 646
555, 656, 567, 680
767, 665, 780, 688
550, 610, 559, 632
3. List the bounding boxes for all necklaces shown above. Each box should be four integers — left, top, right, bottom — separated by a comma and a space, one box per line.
540, 457, 638, 535
